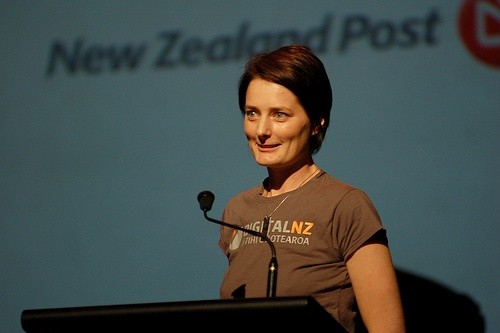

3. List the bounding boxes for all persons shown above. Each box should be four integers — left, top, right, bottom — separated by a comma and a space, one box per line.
217, 45, 405, 333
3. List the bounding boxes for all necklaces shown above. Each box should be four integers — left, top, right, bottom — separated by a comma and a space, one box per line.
260, 164, 319, 242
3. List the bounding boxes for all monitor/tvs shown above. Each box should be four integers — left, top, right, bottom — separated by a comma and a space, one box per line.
20, 295, 349, 333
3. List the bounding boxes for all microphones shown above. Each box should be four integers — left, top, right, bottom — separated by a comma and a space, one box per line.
197, 191, 278, 299
